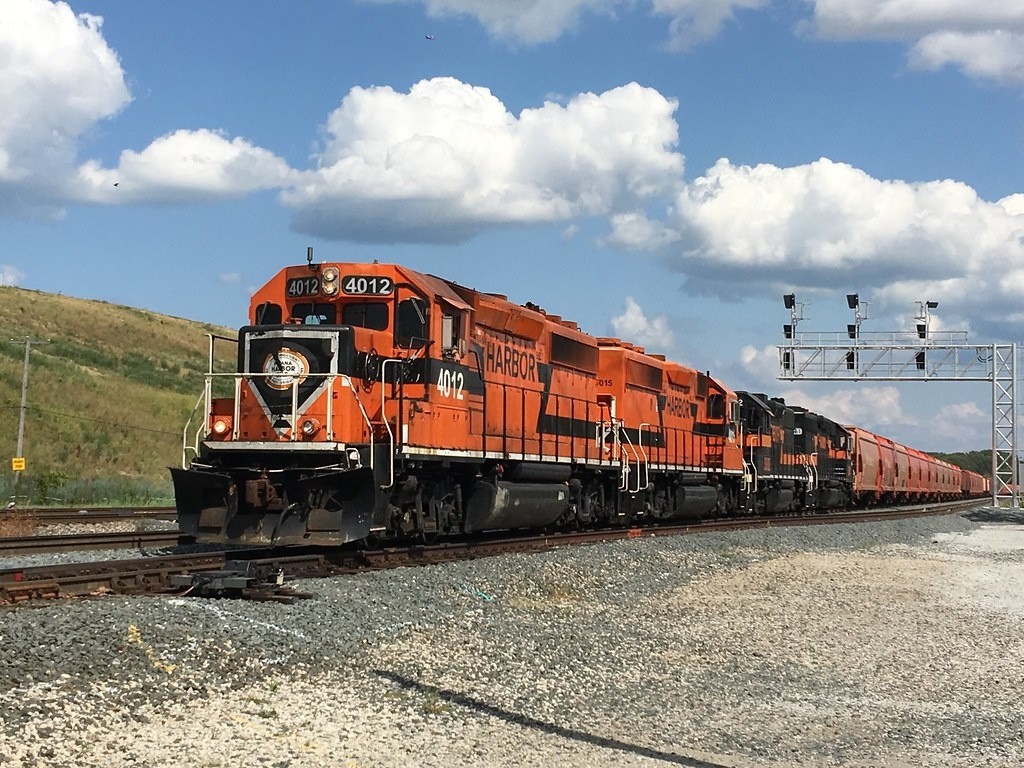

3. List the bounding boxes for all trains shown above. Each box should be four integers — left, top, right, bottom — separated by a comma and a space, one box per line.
166, 246, 1024, 552
